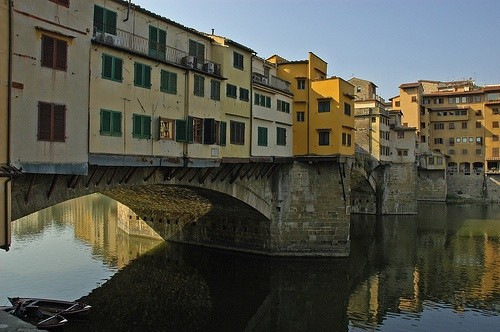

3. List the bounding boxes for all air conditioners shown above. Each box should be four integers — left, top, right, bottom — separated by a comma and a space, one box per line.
95, 31, 116, 44
183, 55, 216, 73
252, 73, 268, 84
